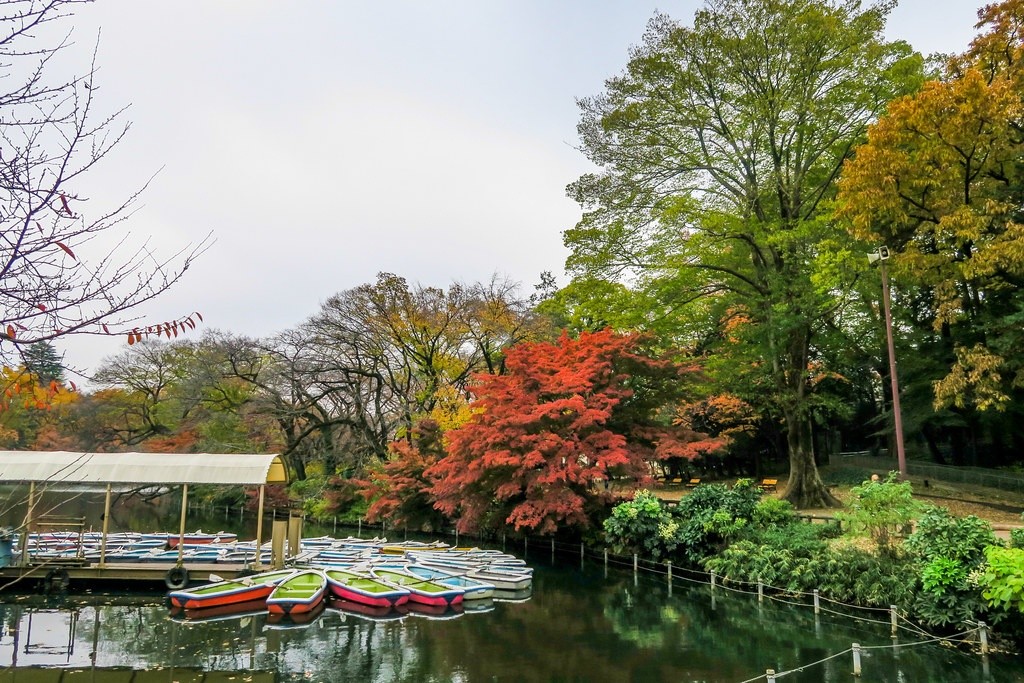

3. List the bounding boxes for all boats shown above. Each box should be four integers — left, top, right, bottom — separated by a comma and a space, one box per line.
404, 564, 495, 600
166, 596, 269, 625
369, 566, 465, 606
267, 597, 327, 630
322, 567, 411, 607
10, 528, 534, 591
265, 569, 328, 612
410, 601, 465, 621
169, 568, 298, 610
327, 592, 410, 622
462, 599, 496, 615
492, 590, 533, 604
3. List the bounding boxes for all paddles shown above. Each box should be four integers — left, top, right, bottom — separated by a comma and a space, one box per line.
433, 570, 475, 582
244, 576, 257, 585
306, 534, 493, 573
10, 527, 305, 565
349, 574, 389, 581
265, 579, 291, 589
209, 573, 248, 587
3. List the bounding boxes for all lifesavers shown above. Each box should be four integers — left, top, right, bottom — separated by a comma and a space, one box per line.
44, 570, 69, 582
165, 566, 189, 590
